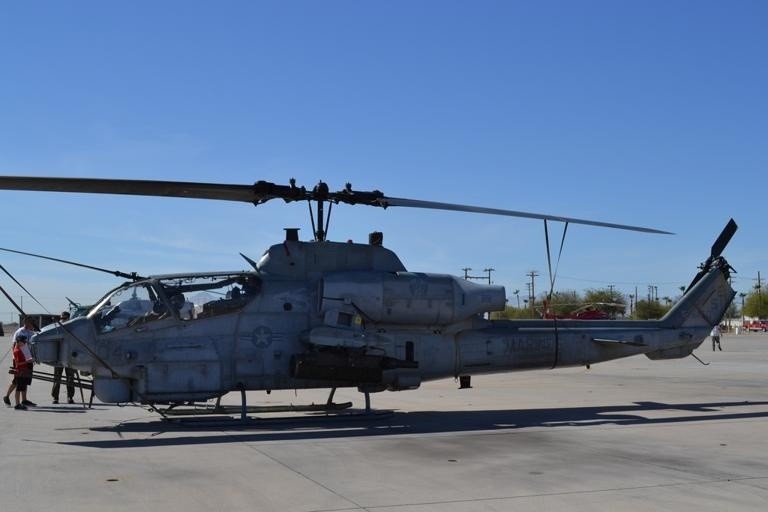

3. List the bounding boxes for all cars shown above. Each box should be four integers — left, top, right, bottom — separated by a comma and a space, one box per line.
744, 322, 768, 332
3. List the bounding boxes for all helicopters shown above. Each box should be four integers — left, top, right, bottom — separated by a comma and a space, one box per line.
1, 246, 230, 328
531, 299, 628, 320
0, 173, 738, 427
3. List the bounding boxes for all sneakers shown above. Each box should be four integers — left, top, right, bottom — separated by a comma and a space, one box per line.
22, 399, 37, 407
15, 404, 26, 409
67, 397, 74, 403
4, 396, 11, 405
53, 400, 58, 403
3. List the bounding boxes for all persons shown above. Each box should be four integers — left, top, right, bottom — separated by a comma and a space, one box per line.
50, 311, 75, 404
710, 325, 723, 352
2, 315, 37, 407
12, 335, 37, 410
